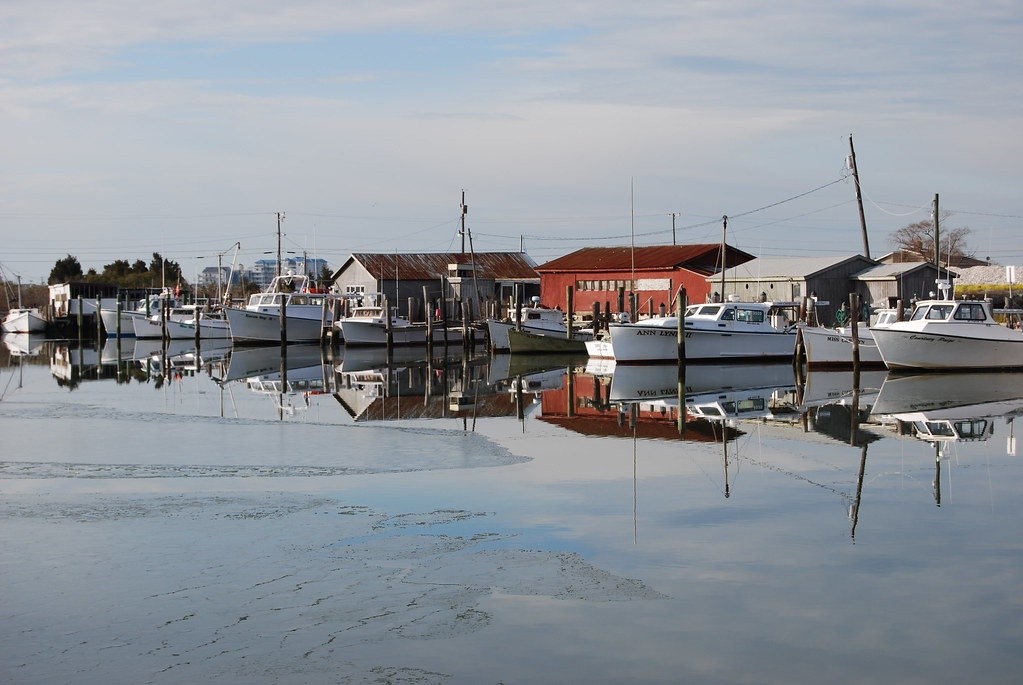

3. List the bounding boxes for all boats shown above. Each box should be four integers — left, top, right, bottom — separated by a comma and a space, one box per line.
857, 371, 1023, 519
488, 354, 590, 435
485, 296, 604, 354
610, 215, 805, 366
869, 232, 1023, 370
799, 235, 954, 371
0, 257, 230, 340
1, 333, 45, 401
333, 248, 488, 348
506, 328, 587, 352
585, 329, 615, 360
801, 372, 888, 413
607, 366, 799, 499
221, 223, 364, 348
48, 340, 406, 423
